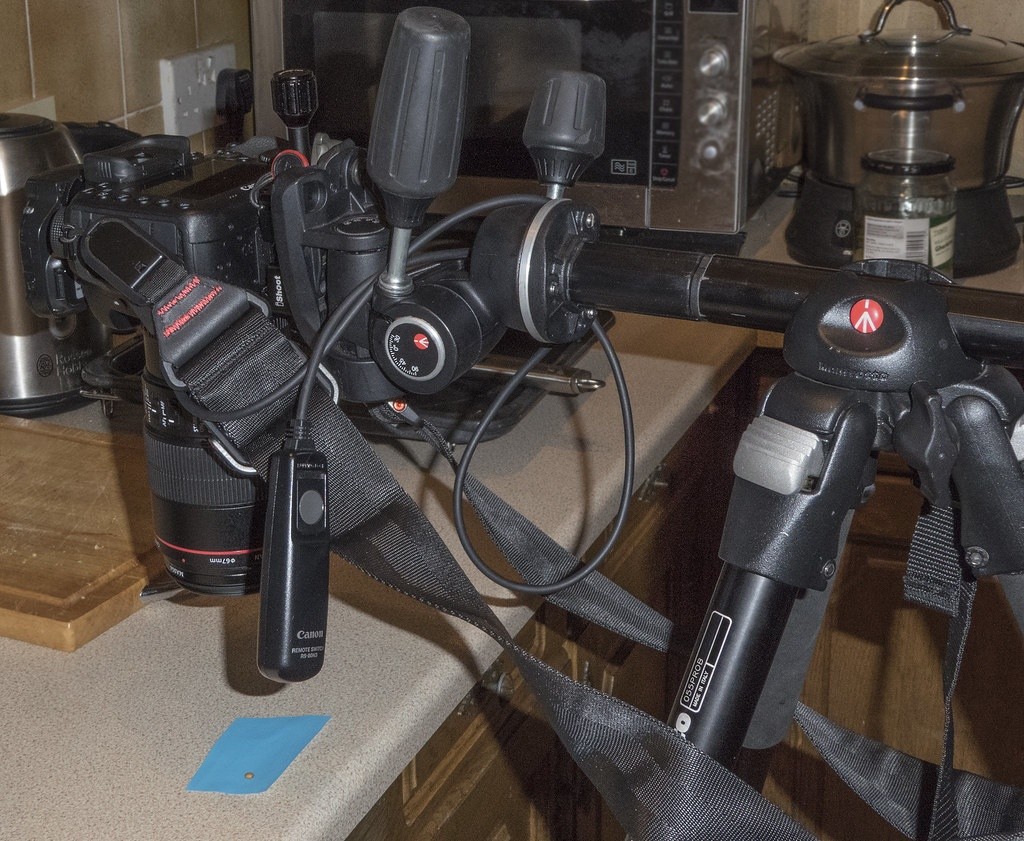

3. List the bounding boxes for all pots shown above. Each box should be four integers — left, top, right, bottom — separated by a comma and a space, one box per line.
767, 0, 1024, 191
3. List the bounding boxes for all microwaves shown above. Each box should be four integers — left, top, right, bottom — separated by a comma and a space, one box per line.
250, 1, 820, 237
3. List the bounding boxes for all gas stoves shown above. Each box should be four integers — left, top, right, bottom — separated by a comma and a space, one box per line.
768, 168, 1023, 279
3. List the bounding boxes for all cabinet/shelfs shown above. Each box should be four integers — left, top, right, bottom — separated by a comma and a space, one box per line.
0, 302, 757, 840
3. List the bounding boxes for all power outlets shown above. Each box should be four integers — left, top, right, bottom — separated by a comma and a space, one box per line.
159, 38, 247, 142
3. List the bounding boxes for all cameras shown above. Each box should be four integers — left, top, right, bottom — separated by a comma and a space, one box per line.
22, 9, 608, 680
